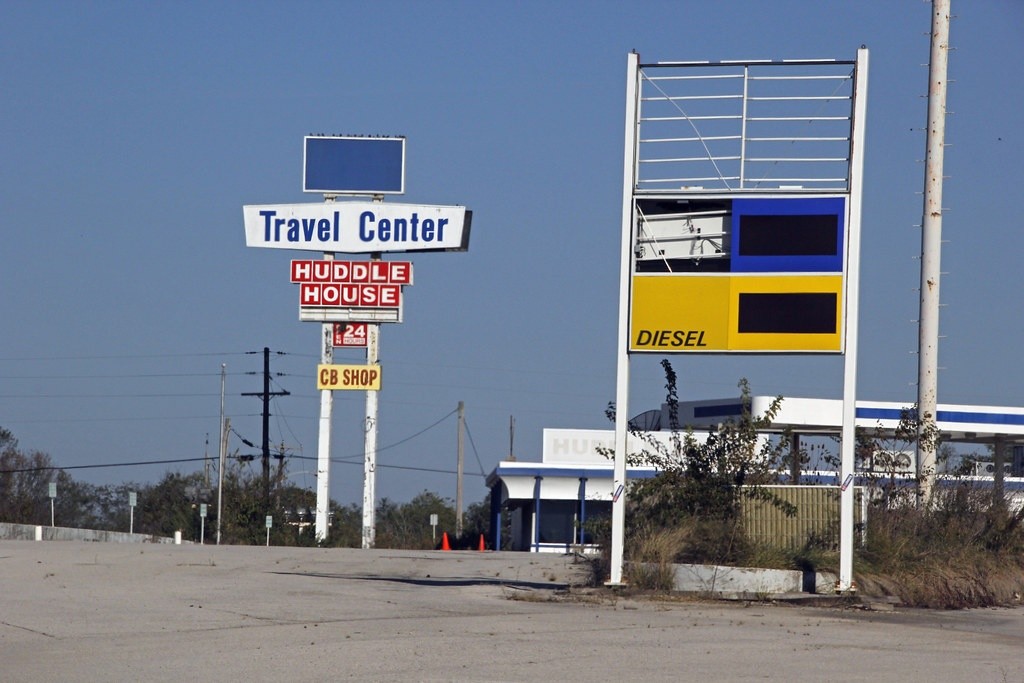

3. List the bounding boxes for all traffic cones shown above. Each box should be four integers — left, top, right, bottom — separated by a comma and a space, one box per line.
478, 534, 486, 550
440, 532, 451, 550
